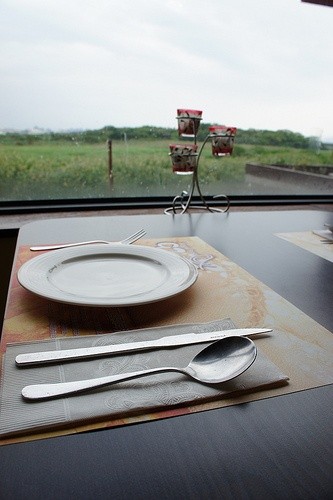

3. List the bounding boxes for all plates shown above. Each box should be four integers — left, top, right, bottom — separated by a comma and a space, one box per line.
16, 244, 199, 306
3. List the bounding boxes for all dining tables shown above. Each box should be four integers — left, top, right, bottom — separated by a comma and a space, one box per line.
0, 211, 333, 500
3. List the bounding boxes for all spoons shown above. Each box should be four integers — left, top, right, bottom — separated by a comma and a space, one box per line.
21, 335, 258, 401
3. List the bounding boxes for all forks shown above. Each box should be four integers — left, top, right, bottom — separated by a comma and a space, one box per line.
30, 228, 147, 251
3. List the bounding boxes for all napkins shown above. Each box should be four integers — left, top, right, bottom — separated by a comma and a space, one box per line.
0, 318, 290, 433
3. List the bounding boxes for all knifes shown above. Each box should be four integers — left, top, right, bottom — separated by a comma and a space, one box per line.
14, 327, 272, 367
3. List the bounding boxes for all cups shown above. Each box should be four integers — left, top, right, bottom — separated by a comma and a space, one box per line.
169, 143, 197, 175
177, 109, 204, 137
208, 125, 237, 157
